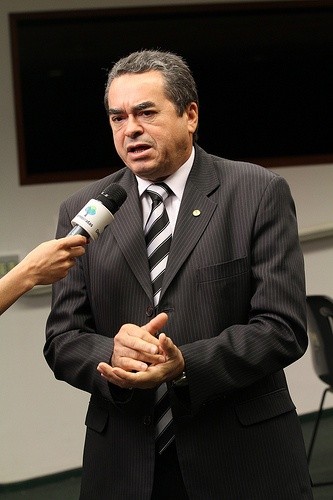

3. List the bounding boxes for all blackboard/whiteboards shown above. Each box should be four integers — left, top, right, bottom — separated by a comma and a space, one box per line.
8, 0, 333, 187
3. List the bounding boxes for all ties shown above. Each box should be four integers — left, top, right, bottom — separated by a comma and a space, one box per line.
145, 182, 185, 455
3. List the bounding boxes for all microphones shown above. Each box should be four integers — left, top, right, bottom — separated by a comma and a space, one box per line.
66, 183, 127, 242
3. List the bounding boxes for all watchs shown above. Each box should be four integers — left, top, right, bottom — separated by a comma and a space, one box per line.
172, 371, 186, 386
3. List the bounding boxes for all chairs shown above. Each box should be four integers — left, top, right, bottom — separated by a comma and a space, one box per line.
304, 295, 333, 487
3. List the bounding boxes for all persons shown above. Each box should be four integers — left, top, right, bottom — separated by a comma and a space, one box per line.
0, 234, 87, 313
42, 52, 314, 500
